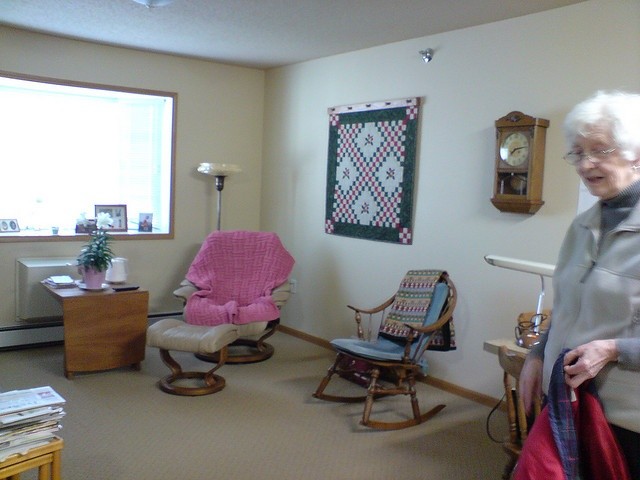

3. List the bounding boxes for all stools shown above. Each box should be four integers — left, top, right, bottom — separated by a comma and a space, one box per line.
146, 318, 241, 396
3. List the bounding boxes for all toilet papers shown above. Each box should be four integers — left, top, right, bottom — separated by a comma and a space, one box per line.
103, 256, 128, 284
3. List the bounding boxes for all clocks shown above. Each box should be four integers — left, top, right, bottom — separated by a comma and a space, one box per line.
499, 131, 531, 169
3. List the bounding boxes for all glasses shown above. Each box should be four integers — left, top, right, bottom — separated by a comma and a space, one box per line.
562, 146, 621, 164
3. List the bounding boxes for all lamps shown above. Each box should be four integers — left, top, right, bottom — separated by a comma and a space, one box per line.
482, 254, 555, 348
197, 161, 241, 228
133, 0, 174, 9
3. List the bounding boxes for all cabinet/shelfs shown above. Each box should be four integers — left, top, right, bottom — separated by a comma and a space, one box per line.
0, 435, 65, 480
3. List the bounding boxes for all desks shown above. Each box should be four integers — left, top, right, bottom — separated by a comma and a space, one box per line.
41, 275, 150, 382
482, 336, 534, 362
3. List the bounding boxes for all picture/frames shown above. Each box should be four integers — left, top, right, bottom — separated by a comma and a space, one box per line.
1, 218, 22, 233
94, 204, 128, 232
138, 213, 153, 232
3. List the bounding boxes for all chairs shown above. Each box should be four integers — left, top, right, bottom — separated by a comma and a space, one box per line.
497, 345, 544, 479
311, 269, 459, 433
172, 230, 296, 362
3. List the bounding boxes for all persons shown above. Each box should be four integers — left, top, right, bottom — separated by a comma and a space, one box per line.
517, 91, 639, 478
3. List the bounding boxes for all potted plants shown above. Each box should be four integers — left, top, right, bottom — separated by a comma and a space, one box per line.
75, 230, 117, 291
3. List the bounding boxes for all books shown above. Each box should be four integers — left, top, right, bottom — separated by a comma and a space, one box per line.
1, 386, 67, 461
45, 276, 78, 289
